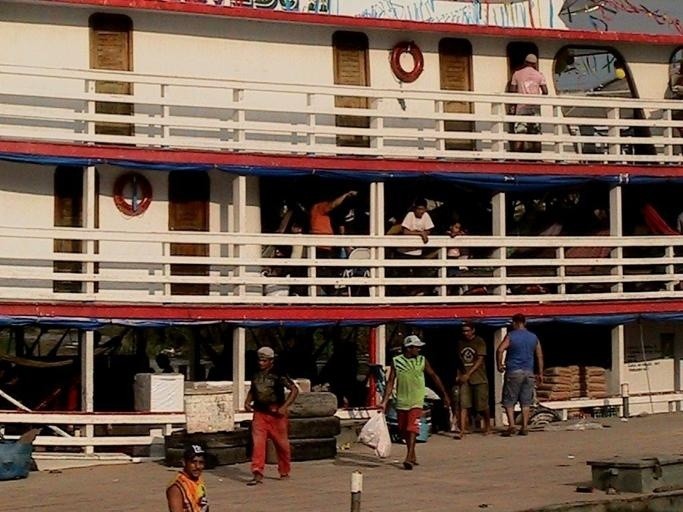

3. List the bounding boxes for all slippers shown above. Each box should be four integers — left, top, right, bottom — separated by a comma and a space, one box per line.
247, 471, 290, 486
454, 426, 530, 440
403, 457, 421, 471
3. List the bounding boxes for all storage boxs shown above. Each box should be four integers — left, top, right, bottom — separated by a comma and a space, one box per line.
133, 373, 184, 413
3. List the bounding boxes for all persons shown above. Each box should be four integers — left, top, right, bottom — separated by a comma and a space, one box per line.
243, 346, 298, 486
506, 53, 548, 153
496, 313, 546, 437
147, 352, 173, 372
454, 322, 492, 438
376, 334, 453, 470
265, 189, 469, 297
164, 444, 212, 512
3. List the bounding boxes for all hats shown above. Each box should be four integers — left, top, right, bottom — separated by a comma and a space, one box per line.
184, 443, 205, 460
403, 334, 425, 347
256, 346, 275, 360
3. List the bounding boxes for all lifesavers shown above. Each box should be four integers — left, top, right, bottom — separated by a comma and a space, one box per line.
392, 38, 424, 84
113, 168, 151, 218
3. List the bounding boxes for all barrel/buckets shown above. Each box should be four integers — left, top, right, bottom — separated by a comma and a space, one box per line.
0, 441, 32, 482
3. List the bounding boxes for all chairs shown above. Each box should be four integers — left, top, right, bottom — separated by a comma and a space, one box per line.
334, 248, 371, 296
